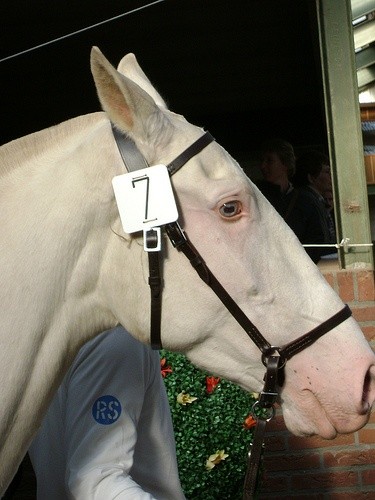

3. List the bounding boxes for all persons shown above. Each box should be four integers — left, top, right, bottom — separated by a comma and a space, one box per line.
27, 324, 189, 499
259, 138, 338, 263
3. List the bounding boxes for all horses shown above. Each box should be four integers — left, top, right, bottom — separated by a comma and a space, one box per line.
0, 44, 375, 500
